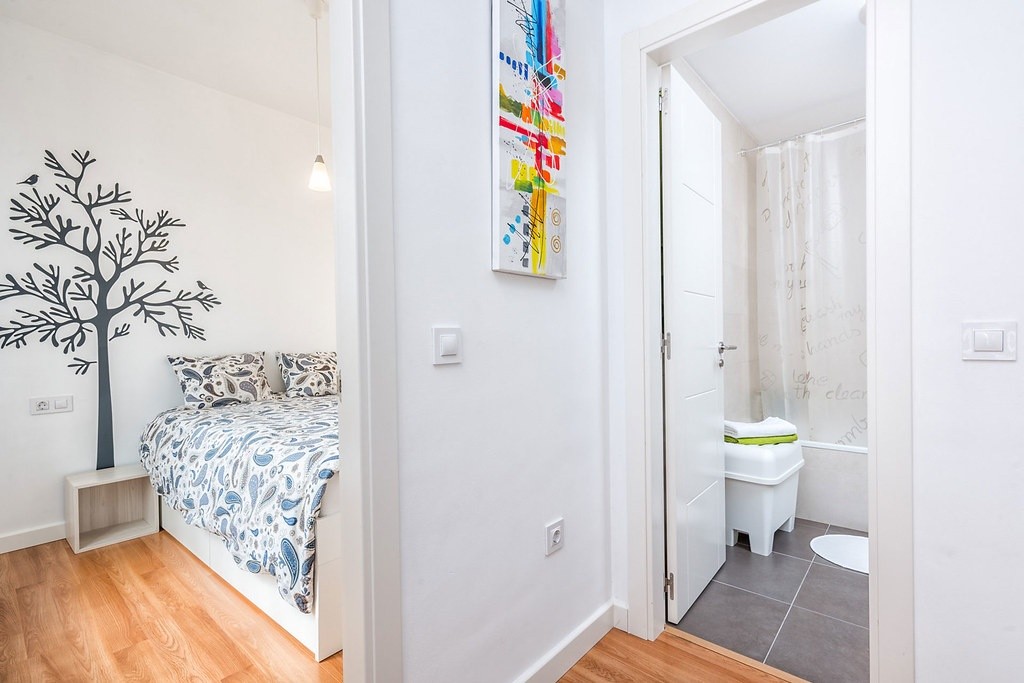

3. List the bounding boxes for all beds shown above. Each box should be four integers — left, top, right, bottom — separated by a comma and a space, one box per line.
137, 392, 343, 663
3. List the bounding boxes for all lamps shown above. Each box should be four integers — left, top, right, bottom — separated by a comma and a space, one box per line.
307, 0, 333, 192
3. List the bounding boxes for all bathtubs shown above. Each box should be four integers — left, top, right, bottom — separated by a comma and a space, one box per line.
796, 440, 868, 532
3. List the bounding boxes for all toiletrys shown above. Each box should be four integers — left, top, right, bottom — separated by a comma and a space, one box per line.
755, 391, 763, 421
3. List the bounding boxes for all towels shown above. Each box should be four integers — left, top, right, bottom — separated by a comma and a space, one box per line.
724, 416, 798, 445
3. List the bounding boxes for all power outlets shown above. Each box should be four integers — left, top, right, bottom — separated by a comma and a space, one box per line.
544, 517, 565, 556
29, 394, 73, 415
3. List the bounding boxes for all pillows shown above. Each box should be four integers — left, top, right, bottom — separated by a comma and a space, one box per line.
166, 350, 278, 410
274, 351, 341, 399
